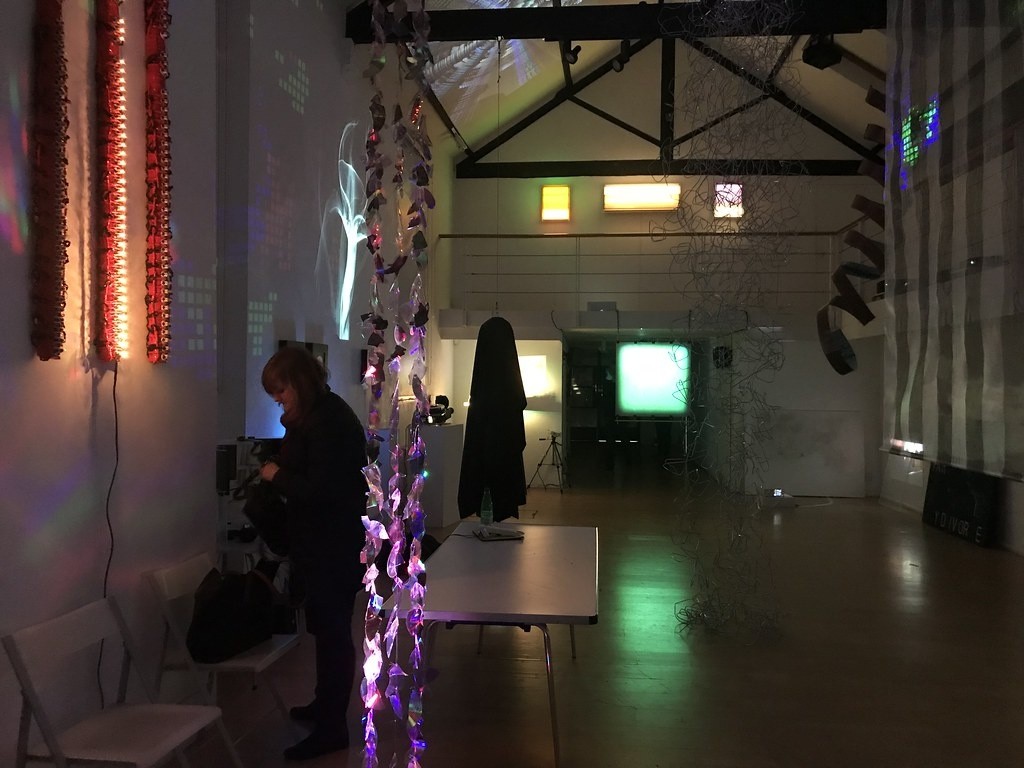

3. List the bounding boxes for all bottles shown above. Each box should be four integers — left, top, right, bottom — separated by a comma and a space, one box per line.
481, 487, 493, 525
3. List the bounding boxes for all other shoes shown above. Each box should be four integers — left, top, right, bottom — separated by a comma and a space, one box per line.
289, 698, 318, 727
282, 728, 349, 761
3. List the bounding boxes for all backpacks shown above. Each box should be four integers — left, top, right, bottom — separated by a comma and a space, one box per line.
184, 570, 270, 665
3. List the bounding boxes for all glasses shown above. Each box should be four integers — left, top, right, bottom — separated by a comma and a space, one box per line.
271, 389, 286, 394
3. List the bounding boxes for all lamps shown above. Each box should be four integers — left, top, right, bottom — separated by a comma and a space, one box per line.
604, 183, 682, 212
612, 40, 631, 73
539, 185, 571, 221
564, 44, 581, 64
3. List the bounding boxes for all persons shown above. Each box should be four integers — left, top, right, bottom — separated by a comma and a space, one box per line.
261, 346, 369, 759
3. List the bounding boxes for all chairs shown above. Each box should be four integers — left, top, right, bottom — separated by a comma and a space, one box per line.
0, 594, 241, 768
145, 554, 300, 768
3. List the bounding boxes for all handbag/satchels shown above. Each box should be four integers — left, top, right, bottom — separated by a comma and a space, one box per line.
242, 478, 306, 558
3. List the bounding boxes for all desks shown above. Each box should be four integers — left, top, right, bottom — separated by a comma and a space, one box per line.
411, 424, 465, 527
380, 521, 601, 768
